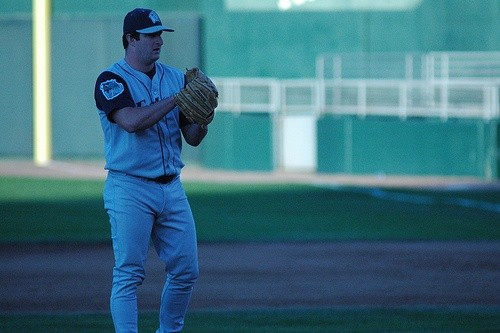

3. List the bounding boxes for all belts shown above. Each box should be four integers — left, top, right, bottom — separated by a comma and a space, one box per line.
133, 175, 173, 184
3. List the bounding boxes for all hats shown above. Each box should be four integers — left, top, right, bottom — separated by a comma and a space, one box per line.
123, 8, 175, 33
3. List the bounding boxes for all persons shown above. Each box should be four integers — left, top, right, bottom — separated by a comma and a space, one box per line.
95, 7, 212, 332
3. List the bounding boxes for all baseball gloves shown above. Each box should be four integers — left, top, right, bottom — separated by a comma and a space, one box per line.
184, 64, 219, 98
173, 78, 219, 126
